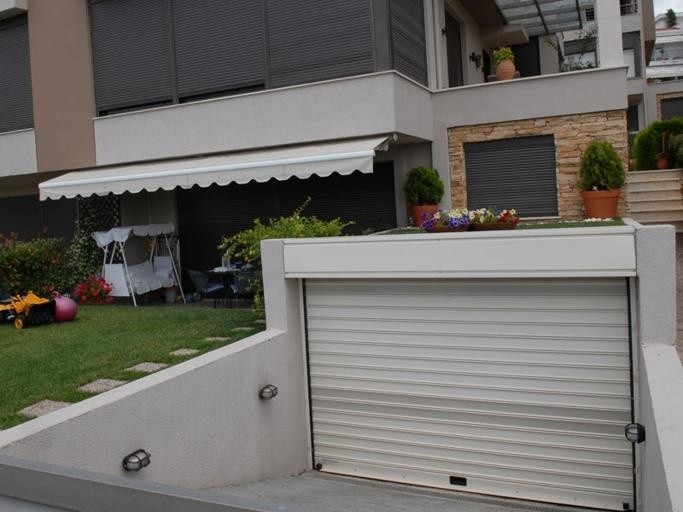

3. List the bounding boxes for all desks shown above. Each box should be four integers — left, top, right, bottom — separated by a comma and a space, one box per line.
206, 268, 241, 305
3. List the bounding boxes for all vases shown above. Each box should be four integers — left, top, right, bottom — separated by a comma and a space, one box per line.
474, 222, 517, 230
427, 226, 467, 234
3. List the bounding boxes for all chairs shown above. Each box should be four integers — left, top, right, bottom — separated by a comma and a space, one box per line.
229, 270, 254, 308
188, 270, 226, 309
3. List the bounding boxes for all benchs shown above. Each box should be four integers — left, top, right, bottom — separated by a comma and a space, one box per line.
91, 223, 177, 307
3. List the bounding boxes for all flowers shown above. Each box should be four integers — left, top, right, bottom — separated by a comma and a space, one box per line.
472, 208, 519, 225
421, 209, 470, 229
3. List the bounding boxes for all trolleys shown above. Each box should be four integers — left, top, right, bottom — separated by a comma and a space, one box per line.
0, 285, 56, 330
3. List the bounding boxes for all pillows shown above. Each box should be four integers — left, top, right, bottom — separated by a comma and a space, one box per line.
156, 264, 173, 279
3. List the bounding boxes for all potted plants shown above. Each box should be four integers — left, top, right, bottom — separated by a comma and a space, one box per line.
493, 44, 516, 80
576, 136, 626, 218
655, 132, 671, 169
407, 165, 443, 226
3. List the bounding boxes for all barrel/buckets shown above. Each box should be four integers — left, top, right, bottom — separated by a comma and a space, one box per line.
166, 288, 176, 303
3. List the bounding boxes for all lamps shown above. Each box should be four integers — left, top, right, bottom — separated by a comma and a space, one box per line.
262, 383, 279, 400
469, 51, 481, 68
122, 449, 150, 470
626, 423, 645, 443
